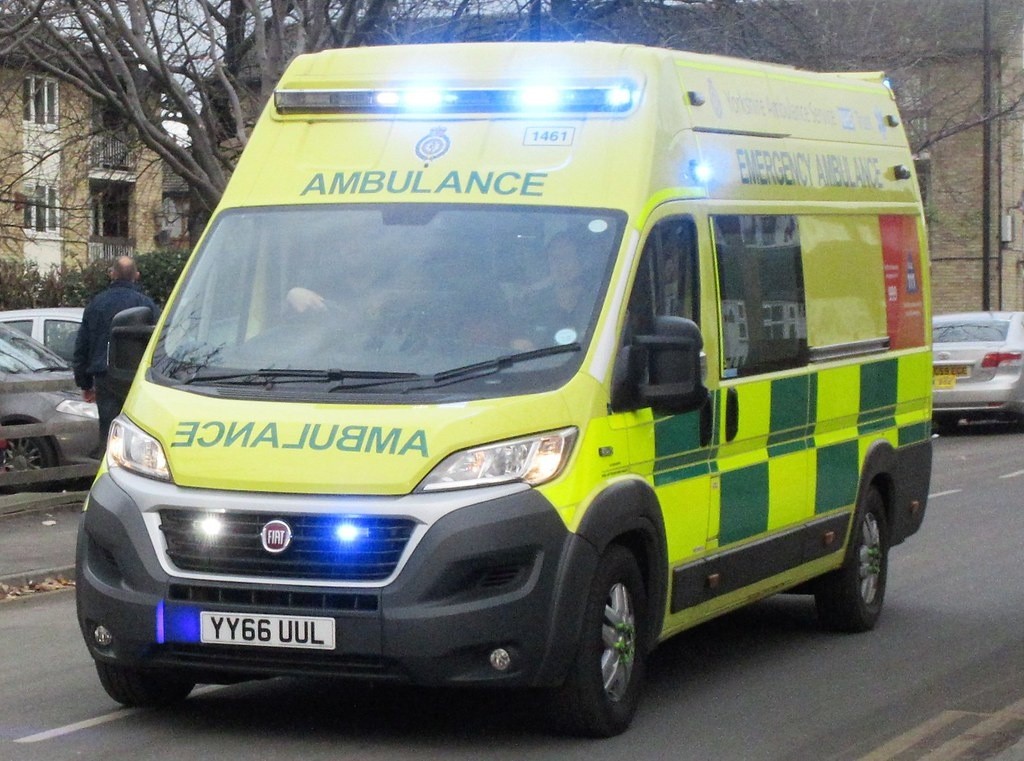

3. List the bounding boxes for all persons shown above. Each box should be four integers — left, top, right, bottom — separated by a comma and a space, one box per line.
274, 237, 381, 323
72, 256, 163, 461
544, 232, 583, 286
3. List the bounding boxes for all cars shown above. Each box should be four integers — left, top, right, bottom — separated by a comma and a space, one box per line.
0, 307, 85, 366
932, 311, 1024, 431
0, 323, 103, 486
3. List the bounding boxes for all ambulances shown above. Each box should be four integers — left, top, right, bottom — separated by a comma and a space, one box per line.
74, 39, 933, 744
